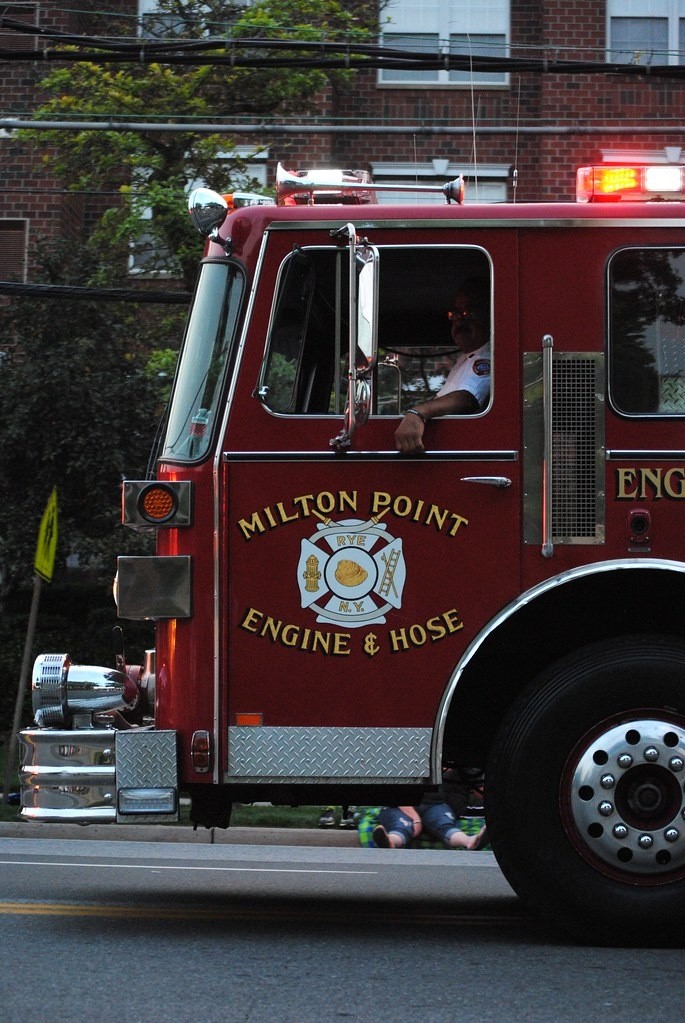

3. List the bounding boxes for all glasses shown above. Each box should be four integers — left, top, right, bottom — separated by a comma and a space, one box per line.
447, 306, 480, 321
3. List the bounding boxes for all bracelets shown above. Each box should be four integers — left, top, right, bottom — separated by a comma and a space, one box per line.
406, 409, 427, 423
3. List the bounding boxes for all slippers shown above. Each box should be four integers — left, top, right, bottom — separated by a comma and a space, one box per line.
472, 829, 490, 851
372, 825, 389, 848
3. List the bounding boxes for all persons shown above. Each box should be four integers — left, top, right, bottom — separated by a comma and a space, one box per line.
373, 804, 489, 851
393, 281, 491, 453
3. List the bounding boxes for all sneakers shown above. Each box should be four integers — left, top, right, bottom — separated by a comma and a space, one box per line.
318, 806, 337, 826
340, 819, 355, 827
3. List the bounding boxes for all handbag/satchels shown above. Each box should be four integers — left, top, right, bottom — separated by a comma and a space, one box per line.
357, 808, 382, 848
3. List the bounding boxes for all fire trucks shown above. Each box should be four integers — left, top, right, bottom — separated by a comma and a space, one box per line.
1, 55, 685, 950
